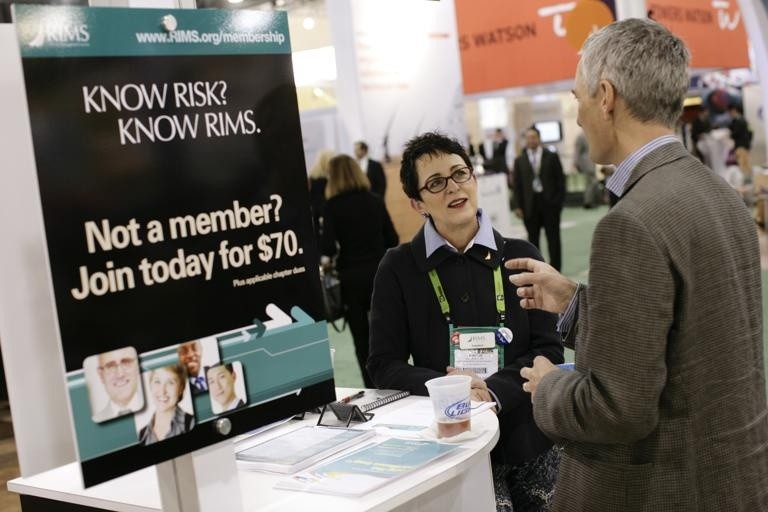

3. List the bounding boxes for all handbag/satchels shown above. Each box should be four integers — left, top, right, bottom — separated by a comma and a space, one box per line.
321, 276, 348, 333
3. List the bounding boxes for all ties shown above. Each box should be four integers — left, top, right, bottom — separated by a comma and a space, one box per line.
194, 377, 205, 392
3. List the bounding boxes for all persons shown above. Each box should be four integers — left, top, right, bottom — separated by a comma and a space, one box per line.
208, 363, 243, 411
504, 19, 768, 512
369, 132, 567, 512
468, 121, 604, 275
690, 107, 719, 164
306, 142, 399, 388
727, 103, 753, 180
176, 342, 211, 396
95, 347, 144, 422
138, 366, 195, 447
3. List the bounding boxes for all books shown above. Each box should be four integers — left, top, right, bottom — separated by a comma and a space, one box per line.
272, 434, 462, 496
347, 388, 410, 411
237, 424, 376, 474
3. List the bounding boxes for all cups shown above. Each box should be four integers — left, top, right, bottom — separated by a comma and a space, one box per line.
425, 375, 473, 439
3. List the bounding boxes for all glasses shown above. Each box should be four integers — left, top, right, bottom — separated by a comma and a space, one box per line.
416, 164, 474, 195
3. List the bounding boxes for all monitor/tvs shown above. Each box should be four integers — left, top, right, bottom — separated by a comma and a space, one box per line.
533, 121, 562, 143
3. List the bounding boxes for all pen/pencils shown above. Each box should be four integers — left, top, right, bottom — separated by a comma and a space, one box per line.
338, 391, 365, 403
